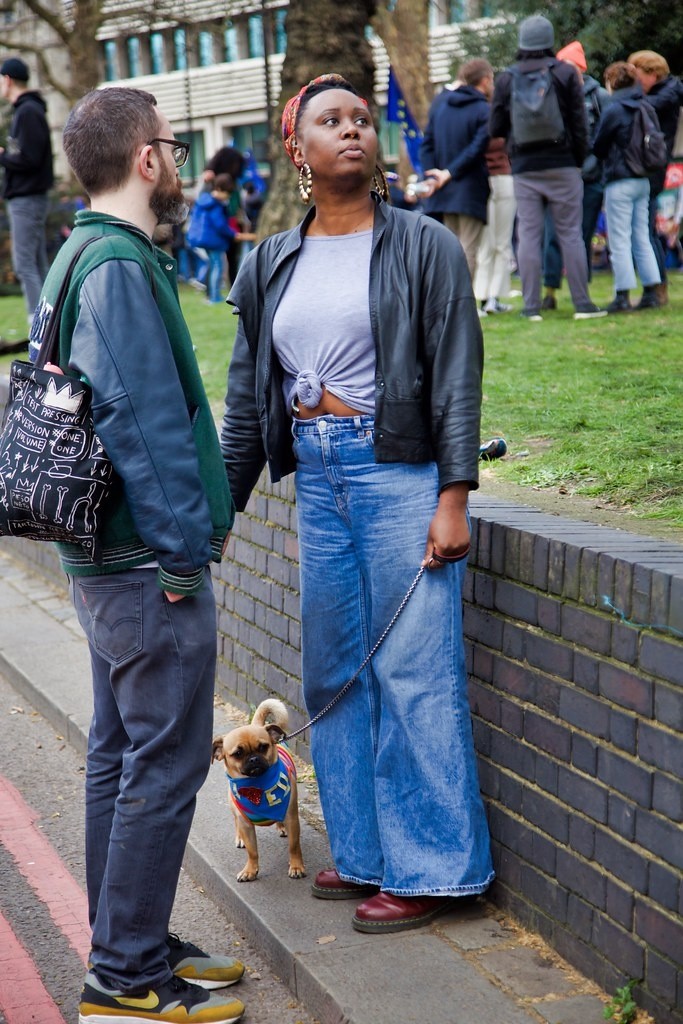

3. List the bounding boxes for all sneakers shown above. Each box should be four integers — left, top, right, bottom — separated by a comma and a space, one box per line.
91, 934, 243, 993
77, 968, 246, 1024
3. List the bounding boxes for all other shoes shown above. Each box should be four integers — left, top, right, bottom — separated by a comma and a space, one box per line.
482, 300, 511, 313
573, 300, 608, 318
542, 296, 556, 310
659, 287, 669, 306
521, 305, 544, 322
631, 294, 658, 311
602, 292, 631, 314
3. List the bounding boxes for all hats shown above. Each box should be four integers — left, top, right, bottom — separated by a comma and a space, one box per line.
517, 15, 555, 51
1, 59, 31, 81
555, 40, 587, 71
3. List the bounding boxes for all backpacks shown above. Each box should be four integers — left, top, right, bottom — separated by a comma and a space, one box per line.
503, 62, 563, 145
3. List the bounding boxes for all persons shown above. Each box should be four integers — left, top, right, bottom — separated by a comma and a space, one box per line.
221, 73, 494, 933
30, 88, 245, 1024
386, 15, 683, 321
0, 57, 55, 326
172, 146, 264, 305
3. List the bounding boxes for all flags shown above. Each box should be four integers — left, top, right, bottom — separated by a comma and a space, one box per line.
387, 71, 425, 175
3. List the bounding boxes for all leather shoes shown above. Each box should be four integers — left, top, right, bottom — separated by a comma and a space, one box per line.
351, 886, 476, 933
311, 867, 380, 900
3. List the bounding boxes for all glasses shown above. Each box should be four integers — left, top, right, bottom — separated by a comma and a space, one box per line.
145, 138, 192, 166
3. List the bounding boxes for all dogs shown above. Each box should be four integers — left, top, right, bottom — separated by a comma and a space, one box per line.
211, 699, 308, 883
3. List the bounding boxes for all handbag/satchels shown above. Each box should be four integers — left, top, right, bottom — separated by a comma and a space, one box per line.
0, 234, 155, 565
633, 103, 668, 174
